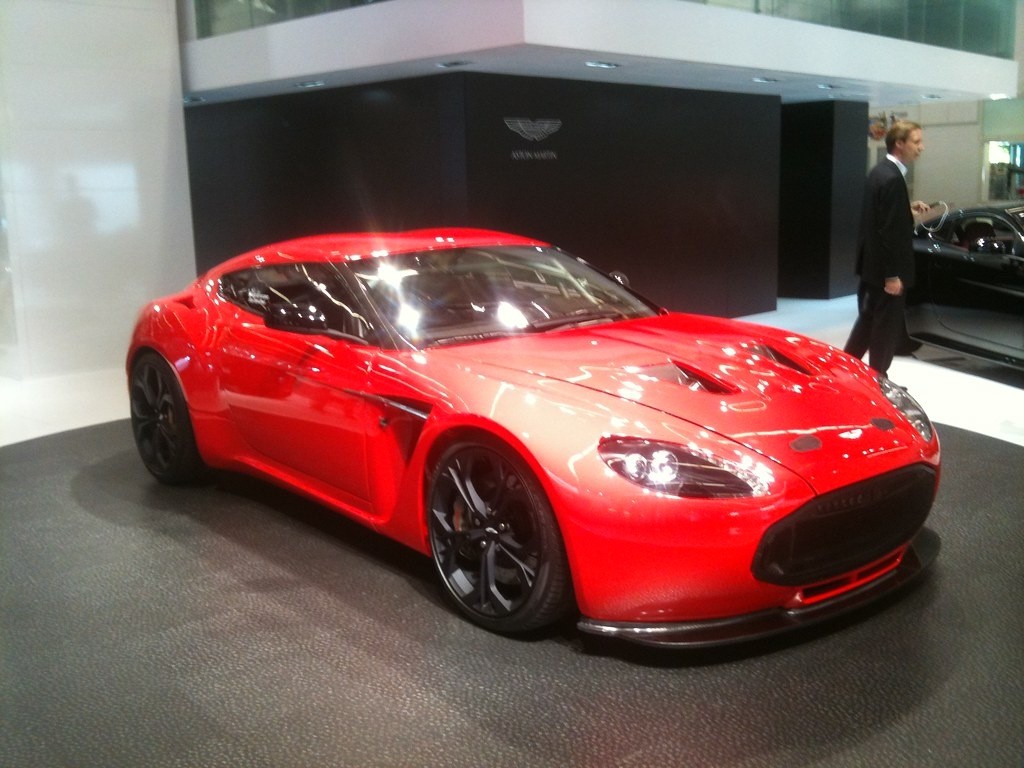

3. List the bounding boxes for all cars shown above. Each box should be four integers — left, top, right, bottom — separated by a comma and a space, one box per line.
911, 200, 1024, 318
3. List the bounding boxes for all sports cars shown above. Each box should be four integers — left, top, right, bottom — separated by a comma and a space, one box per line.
121, 224, 945, 661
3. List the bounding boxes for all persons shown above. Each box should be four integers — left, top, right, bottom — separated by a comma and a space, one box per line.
841, 117, 929, 393
